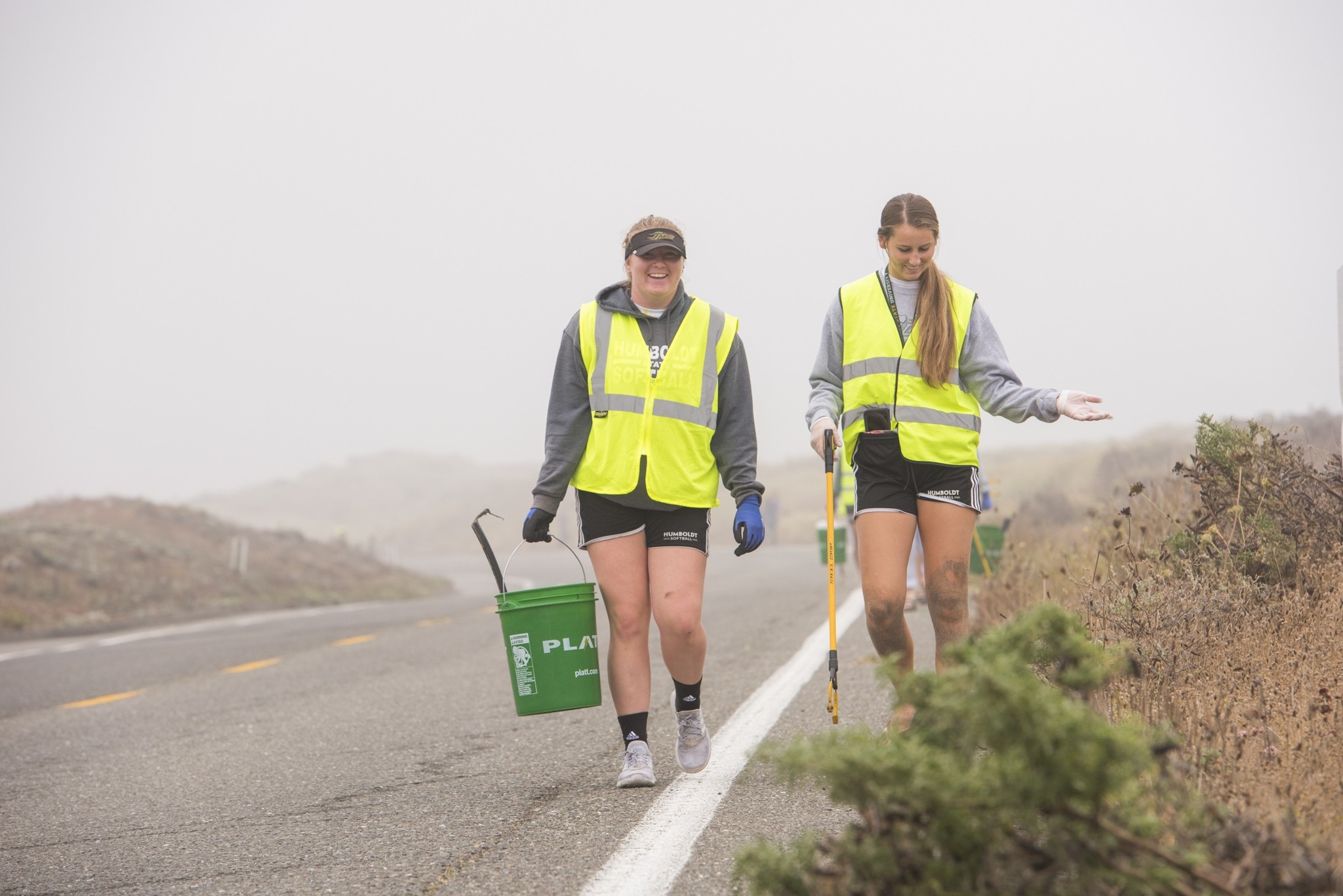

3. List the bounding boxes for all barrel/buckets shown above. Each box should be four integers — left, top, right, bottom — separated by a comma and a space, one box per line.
968, 525, 1005, 574
818, 526, 846, 563
495, 533, 602, 716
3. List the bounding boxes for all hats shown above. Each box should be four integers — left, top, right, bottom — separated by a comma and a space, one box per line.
625, 228, 688, 261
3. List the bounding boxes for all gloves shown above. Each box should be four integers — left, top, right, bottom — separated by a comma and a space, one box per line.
733, 495, 766, 557
522, 508, 555, 543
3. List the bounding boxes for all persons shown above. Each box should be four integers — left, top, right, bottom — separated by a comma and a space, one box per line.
805, 193, 1114, 738
522, 214, 765, 785
897, 523, 928, 612
828, 439, 858, 560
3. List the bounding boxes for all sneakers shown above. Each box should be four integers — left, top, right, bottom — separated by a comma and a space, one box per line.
670, 689, 711, 773
617, 741, 657, 788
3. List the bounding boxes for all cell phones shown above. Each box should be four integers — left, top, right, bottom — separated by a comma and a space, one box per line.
863, 408, 891, 432
